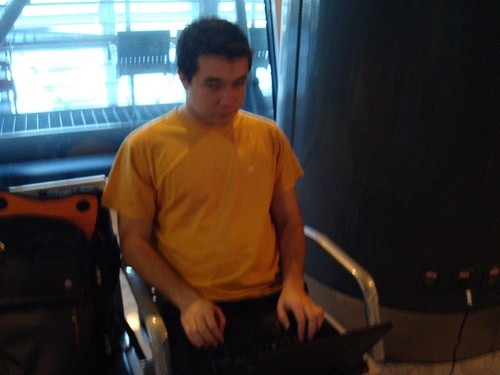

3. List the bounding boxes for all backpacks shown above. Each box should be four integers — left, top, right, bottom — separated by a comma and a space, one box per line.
0, 194, 113, 374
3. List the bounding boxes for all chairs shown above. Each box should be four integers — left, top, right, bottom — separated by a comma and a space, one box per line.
107, 207, 391, 375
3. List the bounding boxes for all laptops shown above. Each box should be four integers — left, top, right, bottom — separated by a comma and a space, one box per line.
186, 312, 393, 375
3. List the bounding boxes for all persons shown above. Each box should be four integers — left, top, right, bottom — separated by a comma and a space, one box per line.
102, 17, 373, 375
